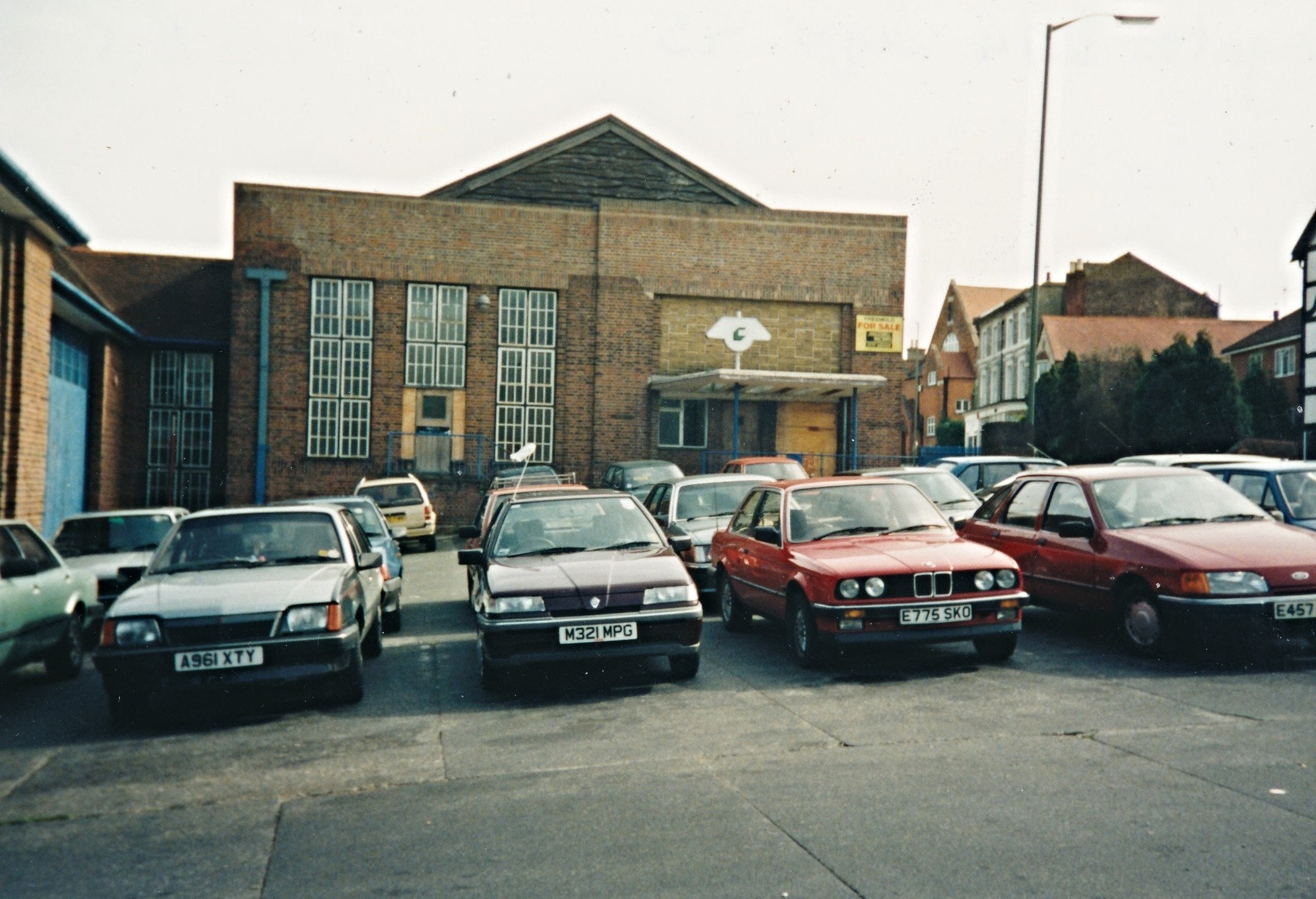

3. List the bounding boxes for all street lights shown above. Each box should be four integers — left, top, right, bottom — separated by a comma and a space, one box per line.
1024, 10, 1161, 458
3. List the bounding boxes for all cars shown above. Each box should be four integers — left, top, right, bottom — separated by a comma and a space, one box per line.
46, 506, 194, 613
640, 472, 803, 598
709, 475, 1029, 661
349, 472, 438, 552
954, 461, 1315, 663
802, 465, 984, 532
602, 458, 686, 506
457, 465, 613, 612
91, 500, 385, 725
1, 517, 106, 684
457, 490, 705, 692
236, 493, 407, 632
924, 453, 1316, 551
716, 457, 809, 482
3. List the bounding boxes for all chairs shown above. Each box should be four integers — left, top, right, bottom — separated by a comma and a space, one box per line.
293, 526, 336, 557
508, 520, 554, 554
397, 485, 412, 500
